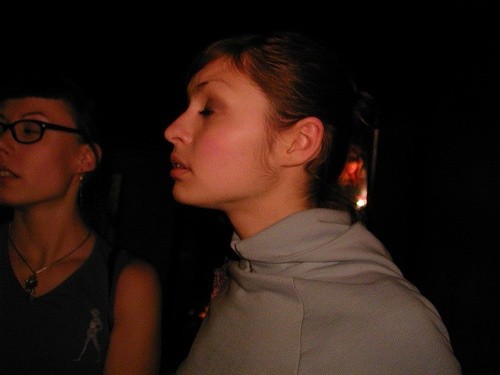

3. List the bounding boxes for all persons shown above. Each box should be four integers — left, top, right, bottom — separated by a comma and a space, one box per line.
0, 79, 161, 374
161, 33, 462, 374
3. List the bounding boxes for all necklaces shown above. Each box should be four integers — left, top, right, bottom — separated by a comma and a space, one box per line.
4, 228, 91, 297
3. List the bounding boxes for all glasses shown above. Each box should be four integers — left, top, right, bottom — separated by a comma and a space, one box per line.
0, 119, 82, 145
